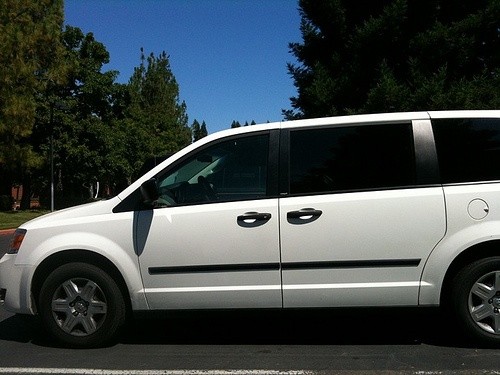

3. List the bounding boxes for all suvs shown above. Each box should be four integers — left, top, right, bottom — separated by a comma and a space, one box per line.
0, 109, 499, 348
